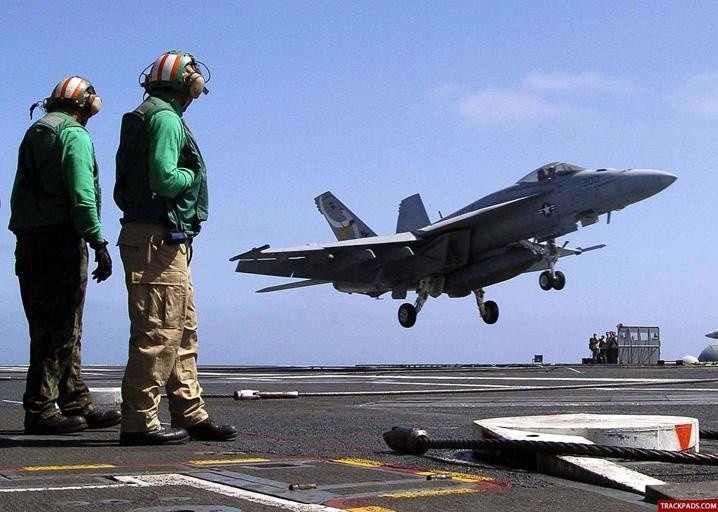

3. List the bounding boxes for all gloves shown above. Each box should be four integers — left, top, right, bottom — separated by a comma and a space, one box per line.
92, 241, 111, 283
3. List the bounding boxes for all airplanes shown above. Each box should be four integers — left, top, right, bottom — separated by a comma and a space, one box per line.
227, 162, 680, 328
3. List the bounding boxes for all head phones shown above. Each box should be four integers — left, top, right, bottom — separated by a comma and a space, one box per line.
142, 53, 206, 99
43, 94, 101, 117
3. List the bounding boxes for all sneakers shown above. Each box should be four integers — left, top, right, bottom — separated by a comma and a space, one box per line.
120, 424, 189, 446
24, 414, 88, 435
171, 418, 240, 441
62, 411, 122, 429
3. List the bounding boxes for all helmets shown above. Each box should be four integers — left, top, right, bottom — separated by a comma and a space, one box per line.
145, 51, 205, 112
42, 76, 101, 127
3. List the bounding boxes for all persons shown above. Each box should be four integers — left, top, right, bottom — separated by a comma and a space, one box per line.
6, 73, 121, 436
112, 51, 240, 444
588, 330, 618, 364
